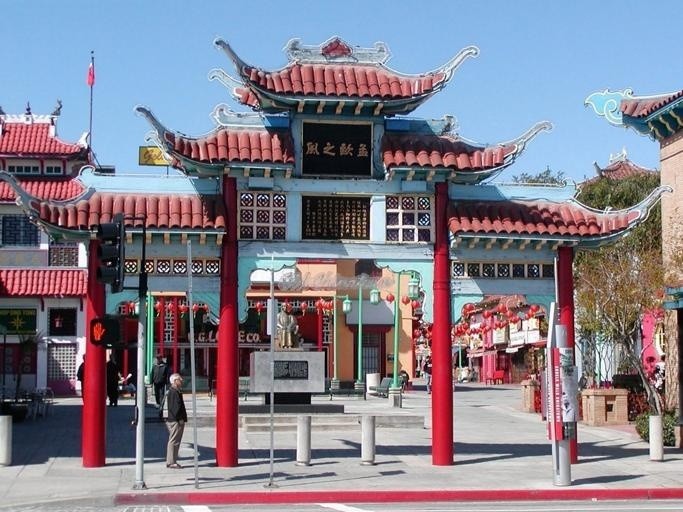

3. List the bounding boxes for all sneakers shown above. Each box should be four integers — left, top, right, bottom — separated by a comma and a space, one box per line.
166, 462, 185, 469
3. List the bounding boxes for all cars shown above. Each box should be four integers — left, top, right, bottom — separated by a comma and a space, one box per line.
457, 368, 472, 382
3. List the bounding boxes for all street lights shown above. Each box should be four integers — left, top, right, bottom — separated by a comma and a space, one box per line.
352, 283, 380, 394
457, 337, 462, 383
390, 270, 421, 408
331, 293, 353, 391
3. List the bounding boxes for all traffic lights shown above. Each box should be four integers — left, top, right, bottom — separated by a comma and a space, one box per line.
95, 222, 118, 282
89, 316, 111, 346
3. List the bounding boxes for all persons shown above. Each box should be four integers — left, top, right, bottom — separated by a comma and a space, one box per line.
158, 371, 188, 469
75, 353, 85, 406
423, 355, 432, 393
150, 352, 165, 407
105, 354, 120, 407
276, 302, 297, 348
398, 370, 409, 394
452, 367, 478, 384
